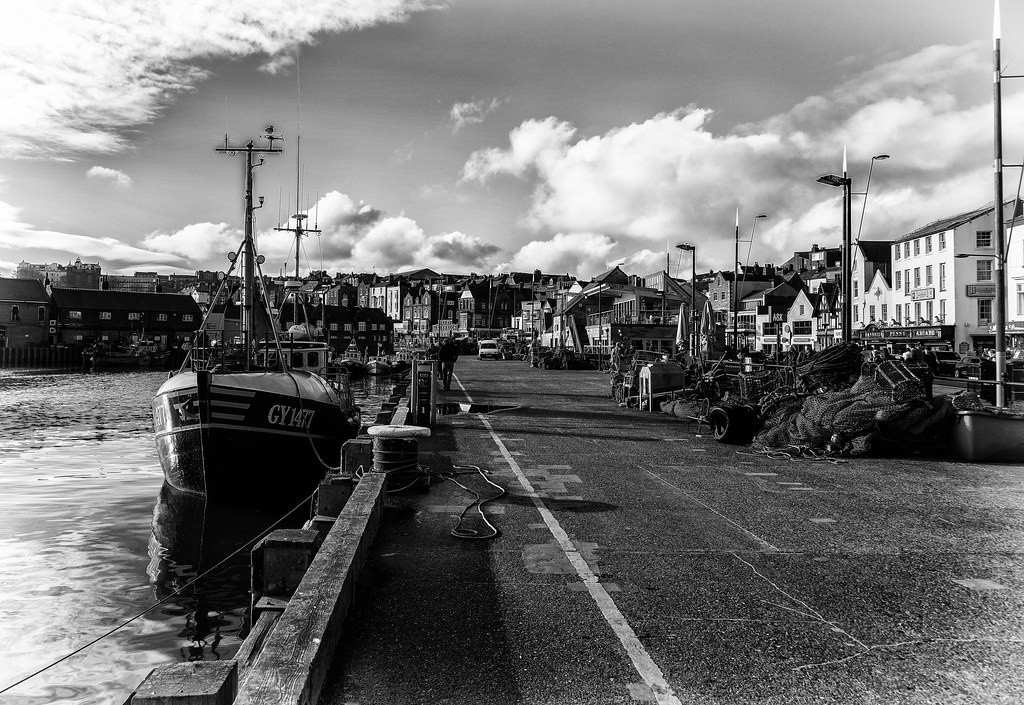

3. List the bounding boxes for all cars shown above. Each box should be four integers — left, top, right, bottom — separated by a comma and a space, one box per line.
954, 356, 995, 380
924, 350, 962, 375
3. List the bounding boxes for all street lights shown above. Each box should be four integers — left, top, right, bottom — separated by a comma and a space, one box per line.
531, 282, 540, 339
591, 280, 602, 371
555, 293, 563, 349
956, 253, 1006, 408
842, 154, 888, 343
816, 175, 852, 343
507, 290, 516, 331
437, 290, 452, 345
489, 283, 504, 340
734, 215, 767, 361
677, 244, 694, 355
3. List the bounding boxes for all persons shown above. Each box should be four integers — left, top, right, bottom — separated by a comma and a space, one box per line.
749, 345, 755, 352
869, 342, 936, 398
783, 345, 816, 364
982, 348, 1011, 407
553, 343, 569, 370
499, 338, 537, 367
759, 349, 767, 370
611, 342, 635, 375
427, 339, 459, 390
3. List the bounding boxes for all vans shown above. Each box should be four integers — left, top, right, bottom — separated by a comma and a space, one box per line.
478, 340, 500, 361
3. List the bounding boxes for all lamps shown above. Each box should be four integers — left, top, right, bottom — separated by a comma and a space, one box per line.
217, 271, 227, 281
228, 251, 237, 262
964, 322, 969, 326
256, 254, 265, 264
210, 339, 219, 348
252, 196, 264, 210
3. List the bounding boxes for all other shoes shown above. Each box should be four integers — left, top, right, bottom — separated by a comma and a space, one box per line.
446, 388, 451, 391
444, 388, 446, 391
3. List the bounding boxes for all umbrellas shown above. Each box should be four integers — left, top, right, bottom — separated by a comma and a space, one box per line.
676, 303, 690, 350
701, 300, 716, 350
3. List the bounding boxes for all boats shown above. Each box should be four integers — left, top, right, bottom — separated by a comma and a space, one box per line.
82, 325, 170, 373
392, 340, 413, 372
155, 124, 365, 514
149, 497, 331, 661
340, 335, 364, 372
366, 344, 391, 375
